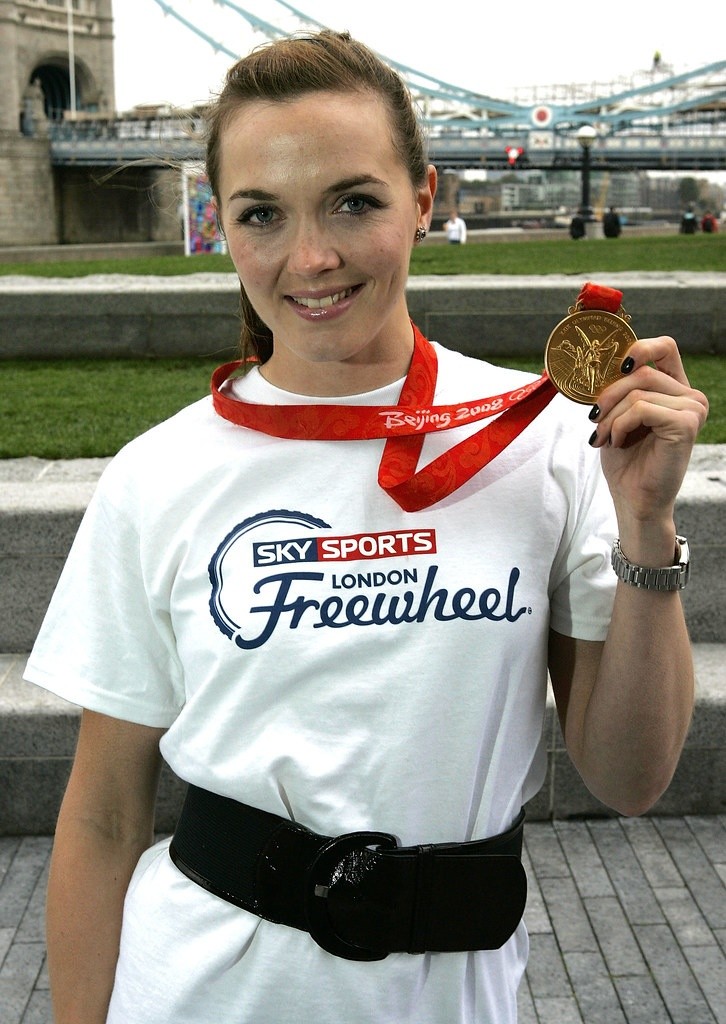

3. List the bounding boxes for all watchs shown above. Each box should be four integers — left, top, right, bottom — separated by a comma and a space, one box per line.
610, 535, 691, 591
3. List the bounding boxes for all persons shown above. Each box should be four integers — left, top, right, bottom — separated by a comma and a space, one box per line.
22, 23, 710, 1023
442, 200, 719, 247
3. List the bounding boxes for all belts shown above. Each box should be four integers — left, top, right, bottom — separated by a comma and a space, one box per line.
169, 786, 528, 962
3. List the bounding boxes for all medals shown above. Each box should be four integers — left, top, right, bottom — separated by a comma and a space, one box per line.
545, 308, 640, 404
